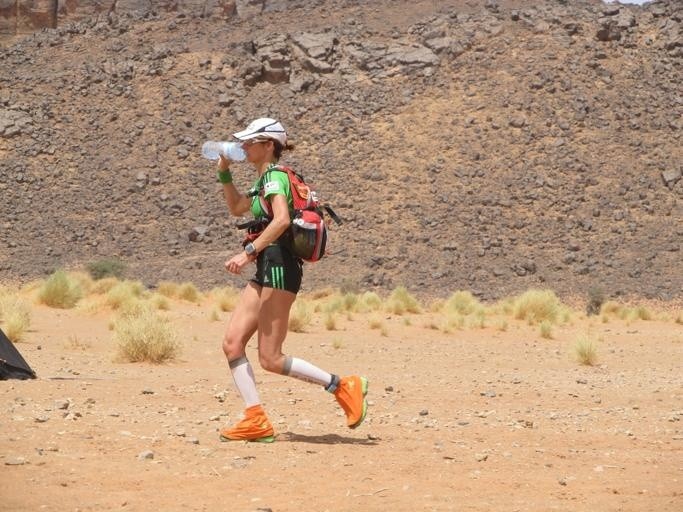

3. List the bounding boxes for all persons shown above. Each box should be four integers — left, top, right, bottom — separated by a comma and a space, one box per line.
215, 115, 369, 444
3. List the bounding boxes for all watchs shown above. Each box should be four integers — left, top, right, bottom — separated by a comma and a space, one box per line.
243, 242, 259, 257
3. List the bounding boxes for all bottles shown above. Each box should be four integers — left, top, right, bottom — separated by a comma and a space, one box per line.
201, 140, 247, 162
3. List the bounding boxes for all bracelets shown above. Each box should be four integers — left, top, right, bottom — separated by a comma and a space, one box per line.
215, 169, 233, 185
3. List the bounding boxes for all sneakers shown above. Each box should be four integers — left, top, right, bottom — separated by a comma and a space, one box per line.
220, 407, 277, 444
334, 376, 369, 429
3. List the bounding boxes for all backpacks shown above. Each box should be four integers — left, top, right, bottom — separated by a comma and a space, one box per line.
286, 166, 332, 262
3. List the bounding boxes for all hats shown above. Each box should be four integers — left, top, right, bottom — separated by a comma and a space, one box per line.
232, 117, 288, 151
